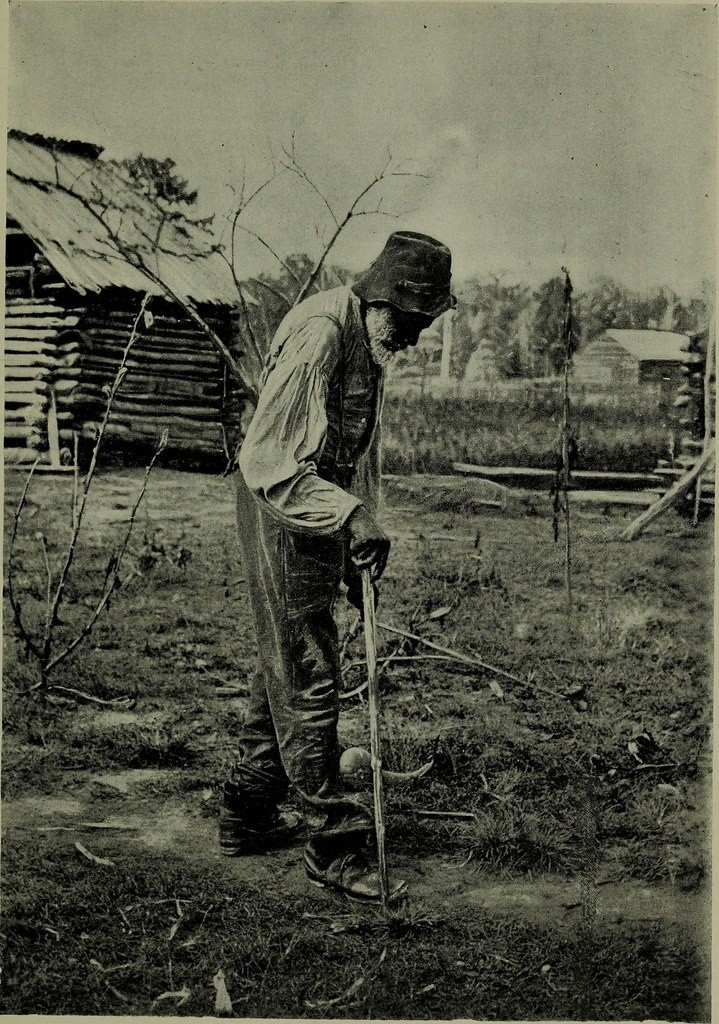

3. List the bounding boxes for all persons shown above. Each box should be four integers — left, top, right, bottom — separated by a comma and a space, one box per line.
220, 232, 457, 903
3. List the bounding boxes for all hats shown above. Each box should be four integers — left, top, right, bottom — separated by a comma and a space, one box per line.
350, 231, 458, 317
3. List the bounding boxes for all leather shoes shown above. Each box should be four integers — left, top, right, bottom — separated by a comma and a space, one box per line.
303, 840, 407, 904
218, 811, 303, 856
